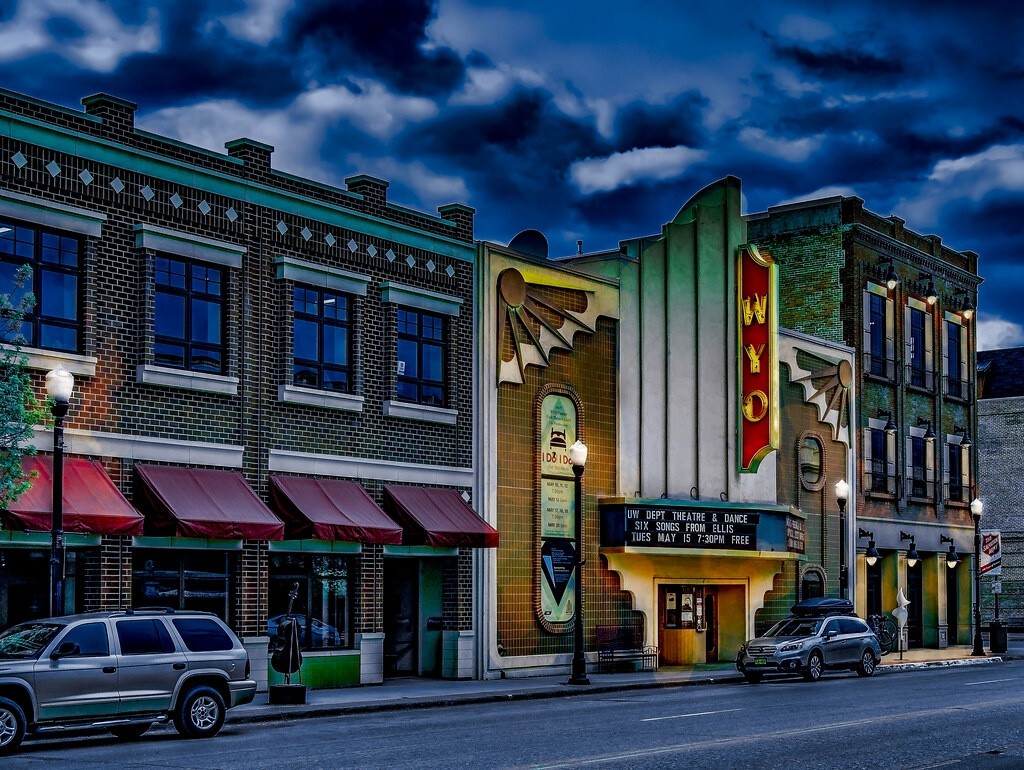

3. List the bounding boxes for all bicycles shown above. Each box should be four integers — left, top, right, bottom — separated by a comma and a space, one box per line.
867, 614, 898, 656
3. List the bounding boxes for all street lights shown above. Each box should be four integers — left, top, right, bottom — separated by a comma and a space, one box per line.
834, 479, 850, 599
971, 498, 988, 657
567, 439, 593, 685
45, 365, 77, 616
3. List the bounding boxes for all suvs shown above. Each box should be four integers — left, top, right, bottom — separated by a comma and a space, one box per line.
736, 597, 881, 685
0, 606, 258, 758
